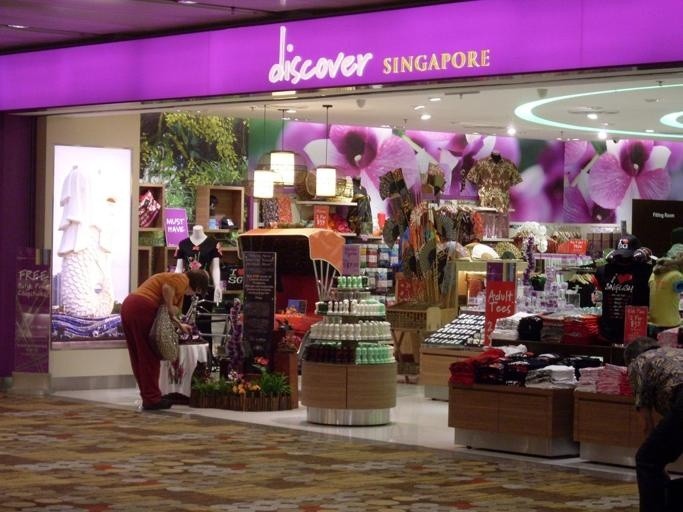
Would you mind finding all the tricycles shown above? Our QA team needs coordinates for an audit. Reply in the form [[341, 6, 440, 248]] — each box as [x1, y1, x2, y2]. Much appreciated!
[[176, 227, 346, 380]]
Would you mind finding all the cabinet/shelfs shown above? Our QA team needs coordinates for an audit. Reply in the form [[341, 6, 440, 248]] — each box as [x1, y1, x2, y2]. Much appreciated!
[[138, 182, 245, 296], [470, 207, 515, 244], [294, 287, 398, 428], [445, 378, 682, 474], [295, 196, 358, 239]]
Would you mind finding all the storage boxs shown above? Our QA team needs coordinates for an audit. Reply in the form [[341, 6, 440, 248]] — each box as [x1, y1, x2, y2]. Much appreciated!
[[357, 242, 399, 289]]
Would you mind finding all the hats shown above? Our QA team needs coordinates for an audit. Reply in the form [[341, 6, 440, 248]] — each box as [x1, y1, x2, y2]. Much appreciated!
[[610, 235, 638, 256], [446, 241, 522, 260]]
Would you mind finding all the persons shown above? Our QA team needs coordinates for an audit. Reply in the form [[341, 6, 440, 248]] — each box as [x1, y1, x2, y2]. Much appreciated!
[[120, 265, 208, 410], [648, 243, 683, 341], [620, 340, 683, 512], [591, 251, 650, 337]]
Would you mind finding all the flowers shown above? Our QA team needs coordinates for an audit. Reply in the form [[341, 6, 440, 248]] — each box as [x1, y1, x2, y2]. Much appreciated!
[[193, 372, 290, 397], [515, 221, 549, 252]]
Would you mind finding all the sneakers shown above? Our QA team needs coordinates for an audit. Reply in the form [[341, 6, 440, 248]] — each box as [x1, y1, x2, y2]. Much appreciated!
[[142, 398, 172, 409]]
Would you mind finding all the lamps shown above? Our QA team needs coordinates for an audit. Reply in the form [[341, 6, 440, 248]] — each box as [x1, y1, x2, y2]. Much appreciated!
[[250, 102, 336, 199]]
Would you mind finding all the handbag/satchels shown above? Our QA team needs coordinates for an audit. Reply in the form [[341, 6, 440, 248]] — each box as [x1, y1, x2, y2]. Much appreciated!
[[147, 304, 179, 363]]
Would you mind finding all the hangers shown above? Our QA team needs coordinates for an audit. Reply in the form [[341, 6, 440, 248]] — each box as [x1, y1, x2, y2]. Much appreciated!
[[544, 221, 581, 245], [569, 264, 598, 286]]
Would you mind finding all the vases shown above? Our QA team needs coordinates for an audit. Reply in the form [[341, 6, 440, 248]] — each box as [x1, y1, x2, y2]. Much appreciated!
[[192, 389, 290, 412]]
